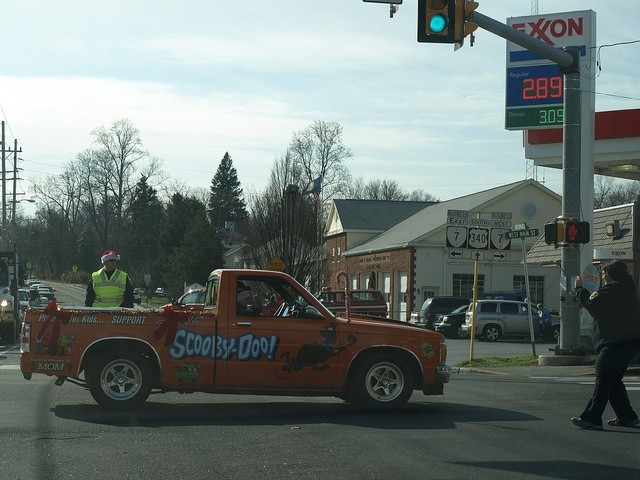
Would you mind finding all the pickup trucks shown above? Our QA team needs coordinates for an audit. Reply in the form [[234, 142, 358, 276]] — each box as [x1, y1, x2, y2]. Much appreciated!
[[20, 269, 451, 410]]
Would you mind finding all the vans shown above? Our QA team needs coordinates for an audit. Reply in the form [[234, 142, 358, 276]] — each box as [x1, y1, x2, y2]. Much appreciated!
[[420, 296, 470, 330], [465, 300, 560, 341], [313, 287, 387, 317], [19, 289, 31, 310]]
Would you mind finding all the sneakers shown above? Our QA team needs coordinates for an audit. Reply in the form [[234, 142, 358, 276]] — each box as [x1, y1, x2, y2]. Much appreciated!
[[571, 417, 603, 431], [607, 418, 640, 428]]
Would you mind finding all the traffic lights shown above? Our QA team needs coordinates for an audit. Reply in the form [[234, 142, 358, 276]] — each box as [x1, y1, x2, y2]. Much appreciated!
[[418, 0, 479, 51], [566, 221, 589, 243], [545, 224, 564, 244]]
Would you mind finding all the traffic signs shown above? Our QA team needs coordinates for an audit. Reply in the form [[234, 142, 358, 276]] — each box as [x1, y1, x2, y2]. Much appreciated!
[[445, 209, 512, 261]]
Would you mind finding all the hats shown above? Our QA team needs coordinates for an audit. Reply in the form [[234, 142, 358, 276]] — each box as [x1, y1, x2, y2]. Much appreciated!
[[101, 249, 120, 263], [236, 281, 250, 294]]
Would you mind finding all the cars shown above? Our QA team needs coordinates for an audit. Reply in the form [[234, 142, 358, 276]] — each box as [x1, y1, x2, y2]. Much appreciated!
[[133, 290, 141, 305], [440, 304, 469, 339], [160, 289, 248, 311]]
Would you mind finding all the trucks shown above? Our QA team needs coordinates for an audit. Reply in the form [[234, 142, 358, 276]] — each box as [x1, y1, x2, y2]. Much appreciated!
[[0, 251, 20, 344]]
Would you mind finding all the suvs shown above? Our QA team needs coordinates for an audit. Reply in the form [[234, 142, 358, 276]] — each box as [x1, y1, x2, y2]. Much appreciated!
[[38, 286, 56, 300]]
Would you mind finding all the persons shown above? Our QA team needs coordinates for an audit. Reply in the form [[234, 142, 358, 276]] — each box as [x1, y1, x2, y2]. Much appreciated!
[[85, 249, 133, 307], [537, 303, 552, 343], [237, 282, 261, 316], [571, 261, 640, 430]]
